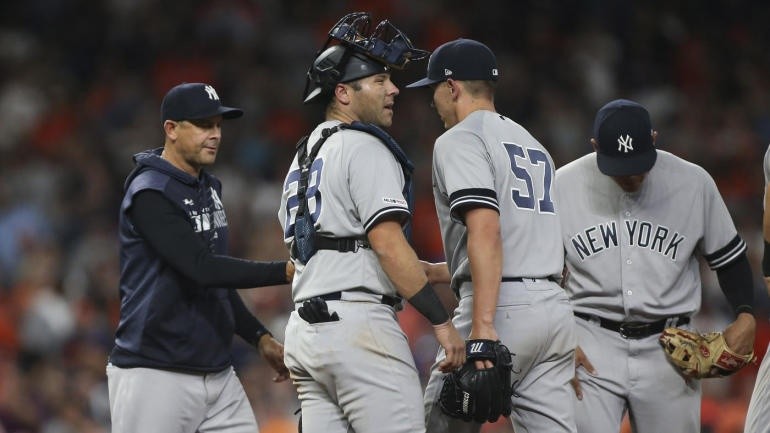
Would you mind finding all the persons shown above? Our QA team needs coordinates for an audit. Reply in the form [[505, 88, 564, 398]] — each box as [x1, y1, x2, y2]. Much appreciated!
[[0, 0, 770, 433], [106, 82, 294, 433]]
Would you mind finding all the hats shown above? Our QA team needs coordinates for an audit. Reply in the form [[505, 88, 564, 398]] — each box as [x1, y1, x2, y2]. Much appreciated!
[[405, 38, 498, 88], [161, 84, 243, 123], [593, 99, 657, 176]]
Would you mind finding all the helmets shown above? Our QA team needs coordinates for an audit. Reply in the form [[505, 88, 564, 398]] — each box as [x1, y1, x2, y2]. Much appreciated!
[[302, 45, 389, 104]]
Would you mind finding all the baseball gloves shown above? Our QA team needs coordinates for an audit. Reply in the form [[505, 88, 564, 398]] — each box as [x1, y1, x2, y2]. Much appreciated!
[[439, 337, 514, 424], [658, 326, 755, 383]]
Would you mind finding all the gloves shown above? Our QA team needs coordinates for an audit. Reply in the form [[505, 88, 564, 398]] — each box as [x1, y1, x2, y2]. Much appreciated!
[[298, 299, 339, 325]]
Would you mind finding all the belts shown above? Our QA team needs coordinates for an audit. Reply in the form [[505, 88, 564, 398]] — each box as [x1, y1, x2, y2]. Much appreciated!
[[321, 292, 401, 306], [455, 276, 556, 300], [574, 311, 690, 340]]
